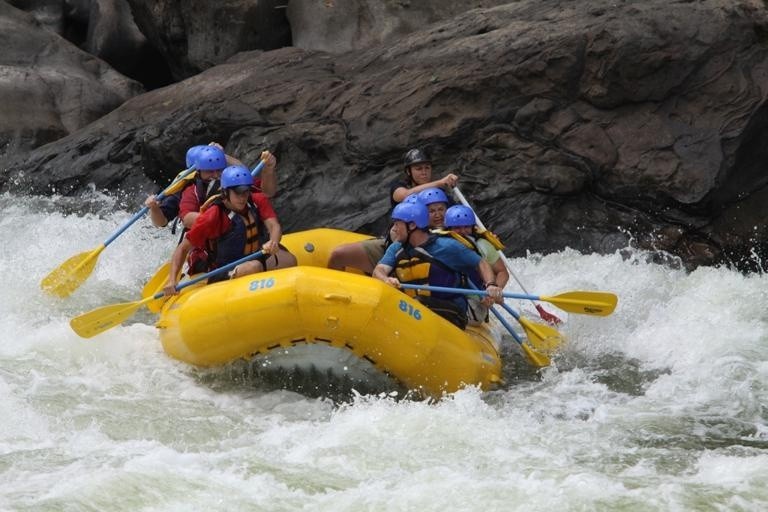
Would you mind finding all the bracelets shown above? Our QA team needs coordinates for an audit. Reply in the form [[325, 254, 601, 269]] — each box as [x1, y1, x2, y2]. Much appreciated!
[[485, 282, 499, 287]]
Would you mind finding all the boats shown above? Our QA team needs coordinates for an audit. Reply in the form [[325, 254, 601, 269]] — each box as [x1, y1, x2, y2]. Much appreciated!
[[146, 216, 512, 401]]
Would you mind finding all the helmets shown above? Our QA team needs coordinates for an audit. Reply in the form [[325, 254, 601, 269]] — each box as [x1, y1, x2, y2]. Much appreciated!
[[444, 205, 476, 228], [221, 165, 254, 189], [404, 148, 431, 167], [392, 203, 429, 228], [186, 144, 227, 171], [401, 188, 448, 205]]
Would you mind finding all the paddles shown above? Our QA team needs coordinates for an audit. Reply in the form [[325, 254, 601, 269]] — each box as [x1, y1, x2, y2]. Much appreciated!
[[399, 283, 616, 316], [40, 165, 197, 299], [452, 186, 566, 368], [70, 251, 268, 338], [142, 160, 266, 313]]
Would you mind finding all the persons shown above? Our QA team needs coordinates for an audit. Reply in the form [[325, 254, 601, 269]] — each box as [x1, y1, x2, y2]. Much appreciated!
[[441, 205, 509, 307], [146, 141, 246, 228], [384, 149, 458, 251], [164, 166, 301, 296], [373, 202, 503, 330], [179, 147, 278, 277], [420, 188, 451, 236]]
